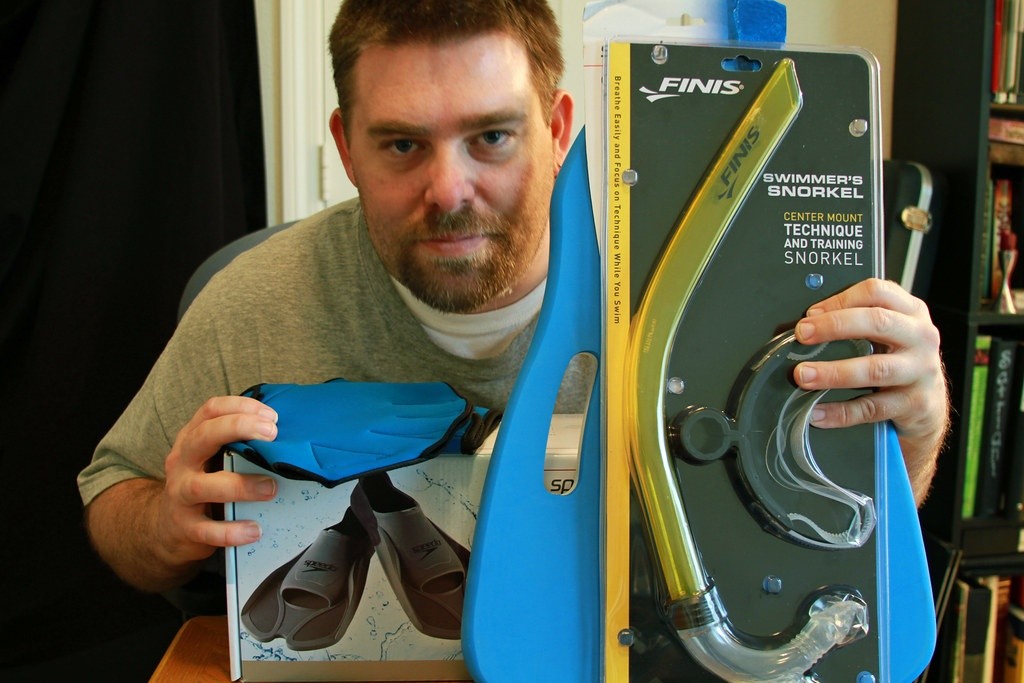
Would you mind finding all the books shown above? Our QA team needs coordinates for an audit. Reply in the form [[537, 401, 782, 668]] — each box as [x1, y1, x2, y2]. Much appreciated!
[[956, 2, 1024, 683]]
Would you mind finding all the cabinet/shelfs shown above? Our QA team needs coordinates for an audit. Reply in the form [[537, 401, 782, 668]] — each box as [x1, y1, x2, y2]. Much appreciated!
[[894, 0, 1024, 575]]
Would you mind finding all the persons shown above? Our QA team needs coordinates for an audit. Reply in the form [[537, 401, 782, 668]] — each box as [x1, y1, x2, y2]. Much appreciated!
[[78, 1, 952, 683]]
